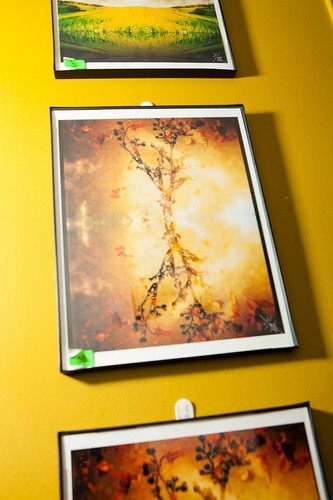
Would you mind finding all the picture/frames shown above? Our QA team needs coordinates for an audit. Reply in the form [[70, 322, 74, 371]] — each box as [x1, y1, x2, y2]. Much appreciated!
[[58, 400, 328, 500], [50, 103, 300, 373], [49, 0, 237, 79]]
